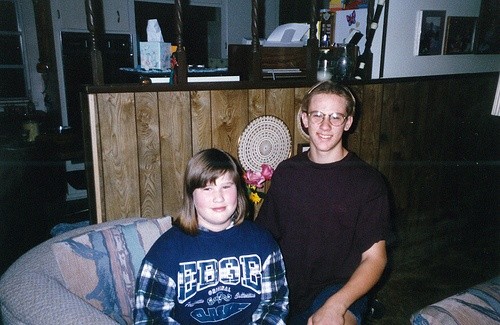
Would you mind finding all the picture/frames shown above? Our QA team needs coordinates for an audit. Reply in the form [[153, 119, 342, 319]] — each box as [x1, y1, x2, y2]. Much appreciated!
[[413, 9, 445, 56], [442, 14, 477, 55], [474, 0, 500, 55]]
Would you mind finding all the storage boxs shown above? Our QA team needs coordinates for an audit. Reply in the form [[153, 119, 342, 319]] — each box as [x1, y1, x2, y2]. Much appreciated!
[[227, 43, 304, 72]]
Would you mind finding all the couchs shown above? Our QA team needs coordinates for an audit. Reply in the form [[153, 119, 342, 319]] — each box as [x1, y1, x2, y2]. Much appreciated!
[[0, 217, 175, 325]]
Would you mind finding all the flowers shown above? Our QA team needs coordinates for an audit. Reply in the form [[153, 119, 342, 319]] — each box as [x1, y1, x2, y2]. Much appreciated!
[[242, 163, 272, 203]]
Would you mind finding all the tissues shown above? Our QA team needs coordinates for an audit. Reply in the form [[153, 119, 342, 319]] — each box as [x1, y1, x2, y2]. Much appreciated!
[[138, 18, 172, 70]]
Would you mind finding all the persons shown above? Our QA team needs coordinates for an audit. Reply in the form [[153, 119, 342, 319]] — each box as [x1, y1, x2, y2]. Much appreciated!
[[255, 83, 391, 325], [135, 149, 289, 325]]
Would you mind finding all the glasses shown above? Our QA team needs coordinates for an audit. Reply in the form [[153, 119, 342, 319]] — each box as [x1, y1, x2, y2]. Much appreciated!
[[308, 110, 348, 126]]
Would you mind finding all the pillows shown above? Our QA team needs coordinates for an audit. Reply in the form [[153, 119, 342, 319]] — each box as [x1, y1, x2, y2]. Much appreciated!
[[54, 214, 174, 324]]
